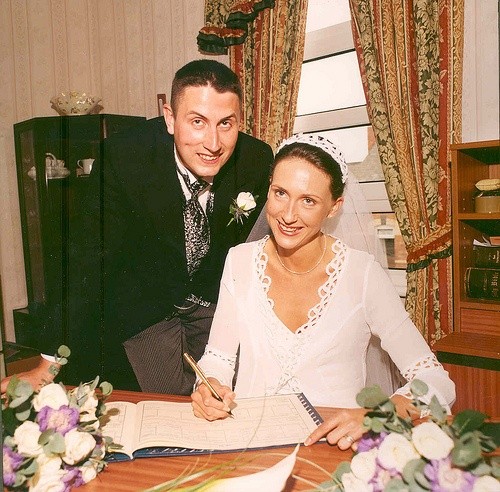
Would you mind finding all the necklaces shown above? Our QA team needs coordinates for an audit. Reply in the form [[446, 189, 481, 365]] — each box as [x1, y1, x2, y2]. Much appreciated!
[[275, 235, 327, 275]]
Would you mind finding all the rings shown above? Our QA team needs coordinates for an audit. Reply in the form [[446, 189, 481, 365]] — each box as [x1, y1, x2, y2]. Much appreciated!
[[344, 433, 355, 444]]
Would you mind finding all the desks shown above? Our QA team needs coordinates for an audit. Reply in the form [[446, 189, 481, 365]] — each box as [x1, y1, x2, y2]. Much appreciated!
[[65, 385, 500, 492]]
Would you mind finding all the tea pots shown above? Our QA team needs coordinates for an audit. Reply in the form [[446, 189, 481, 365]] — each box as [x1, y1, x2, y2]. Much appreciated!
[[44, 153, 57, 168]]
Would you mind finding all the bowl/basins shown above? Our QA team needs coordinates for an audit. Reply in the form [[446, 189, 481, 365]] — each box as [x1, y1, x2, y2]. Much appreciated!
[[50, 91, 102, 116]]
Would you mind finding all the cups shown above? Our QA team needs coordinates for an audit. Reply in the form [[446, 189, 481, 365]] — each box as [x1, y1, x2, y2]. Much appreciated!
[[52, 160, 65, 167], [27, 167, 70, 179], [77, 159, 95, 175]]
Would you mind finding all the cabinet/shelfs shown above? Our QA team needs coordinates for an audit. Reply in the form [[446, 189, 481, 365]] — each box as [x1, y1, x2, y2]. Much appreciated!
[[14, 114, 165, 385], [431, 141, 500, 423]]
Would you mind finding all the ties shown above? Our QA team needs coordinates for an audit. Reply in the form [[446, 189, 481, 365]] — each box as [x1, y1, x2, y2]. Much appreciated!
[[175, 163, 211, 282]]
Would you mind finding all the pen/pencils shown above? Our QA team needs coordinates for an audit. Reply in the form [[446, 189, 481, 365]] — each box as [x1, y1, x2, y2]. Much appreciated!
[[182, 351, 235, 417]]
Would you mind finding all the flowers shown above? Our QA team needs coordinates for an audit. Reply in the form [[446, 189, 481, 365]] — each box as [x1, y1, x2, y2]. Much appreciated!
[[0, 345, 123, 492], [315, 379, 500, 492], [225, 188, 259, 227]]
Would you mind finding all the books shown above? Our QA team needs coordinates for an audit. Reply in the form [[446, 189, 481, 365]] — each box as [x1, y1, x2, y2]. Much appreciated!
[[98, 392, 329, 461], [465, 237, 500, 301]]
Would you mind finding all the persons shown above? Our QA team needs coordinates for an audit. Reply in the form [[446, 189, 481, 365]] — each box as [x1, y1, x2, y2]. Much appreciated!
[[0, 58, 274, 407], [192, 134, 455, 451]]
